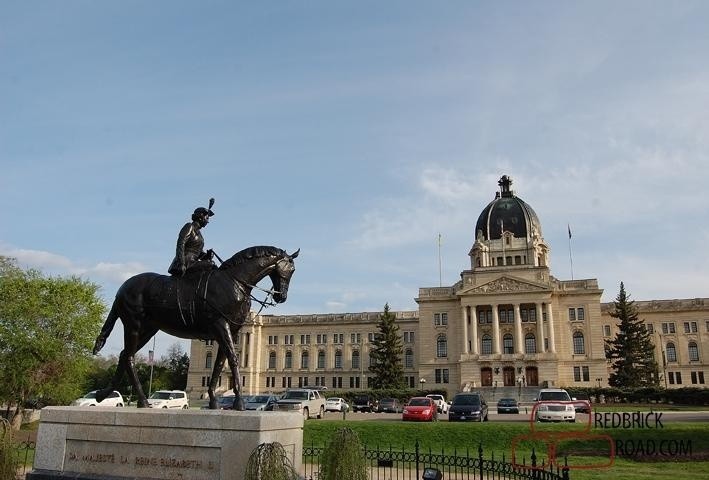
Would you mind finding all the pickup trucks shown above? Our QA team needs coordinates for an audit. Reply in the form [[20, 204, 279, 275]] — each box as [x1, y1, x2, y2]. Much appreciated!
[[571, 394, 591, 415]]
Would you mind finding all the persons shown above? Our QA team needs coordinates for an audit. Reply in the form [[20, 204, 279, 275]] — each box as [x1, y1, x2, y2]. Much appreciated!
[[167, 196, 218, 275]]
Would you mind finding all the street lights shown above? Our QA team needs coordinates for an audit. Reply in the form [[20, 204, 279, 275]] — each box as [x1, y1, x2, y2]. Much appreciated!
[[596, 377, 602, 388], [419, 377, 426, 389]]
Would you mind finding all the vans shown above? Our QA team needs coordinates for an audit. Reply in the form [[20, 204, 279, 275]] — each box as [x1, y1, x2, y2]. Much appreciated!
[[533, 387, 575, 423]]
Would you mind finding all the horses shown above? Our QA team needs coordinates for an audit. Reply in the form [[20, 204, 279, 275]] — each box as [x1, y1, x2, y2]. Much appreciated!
[[92, 246, 300, 411]]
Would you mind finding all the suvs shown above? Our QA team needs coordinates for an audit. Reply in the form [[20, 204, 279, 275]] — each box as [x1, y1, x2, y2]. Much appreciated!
[[273, 387, 327, 419], [74, 389, 125, 407]]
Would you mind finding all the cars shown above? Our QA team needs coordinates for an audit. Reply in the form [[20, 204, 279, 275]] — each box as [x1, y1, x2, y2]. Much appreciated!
[[143, 389, 189, 411], [203, 387, 520, 423]]
